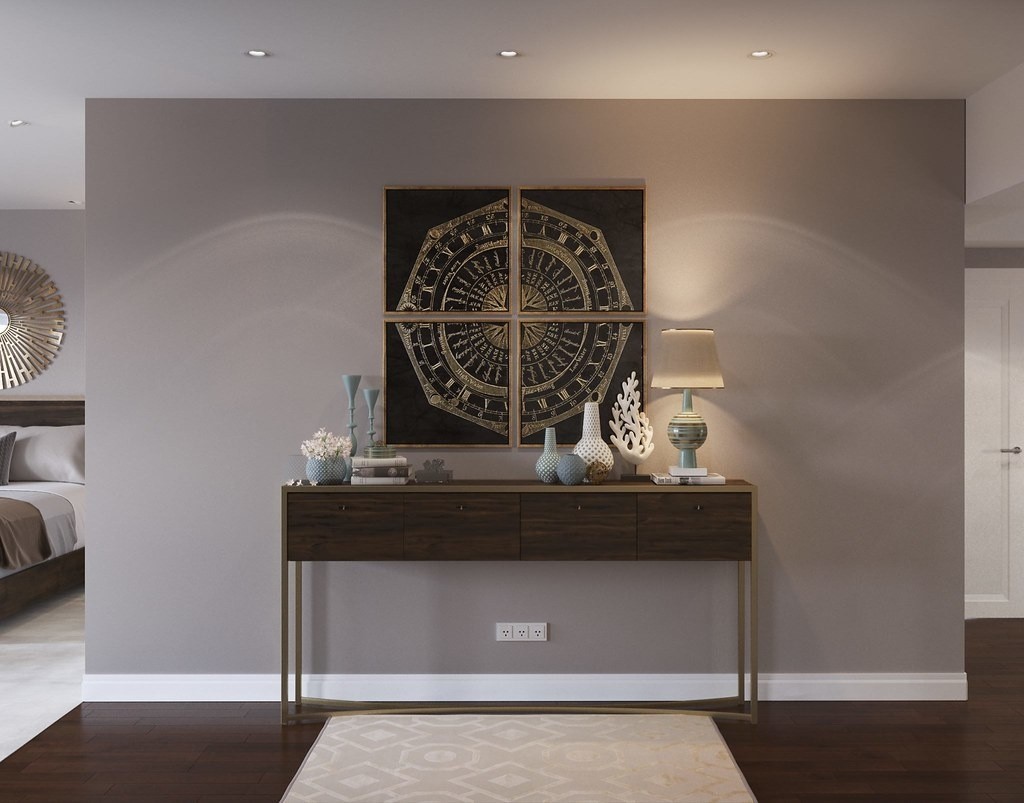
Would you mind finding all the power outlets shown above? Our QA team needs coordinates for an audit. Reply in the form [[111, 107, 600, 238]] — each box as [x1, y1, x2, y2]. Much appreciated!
[[495, 622, 548, 641]]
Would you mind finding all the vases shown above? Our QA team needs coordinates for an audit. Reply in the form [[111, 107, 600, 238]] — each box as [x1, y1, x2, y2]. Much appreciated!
[[363, 388, 380, 448], [341, 374, 362, 481], [557, 454, 587, 484], [572, 401, 615, 483], [535, 428, 562, 483], [306, 455, 347, 485]]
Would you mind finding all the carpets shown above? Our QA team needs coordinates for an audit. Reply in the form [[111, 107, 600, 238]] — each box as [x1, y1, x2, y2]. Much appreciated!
[[280, 714, 761, 803], [0, 581, 86, 763]]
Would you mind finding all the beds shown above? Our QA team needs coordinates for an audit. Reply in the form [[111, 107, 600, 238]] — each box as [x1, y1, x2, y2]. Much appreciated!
[[0, 394, 86, 620]]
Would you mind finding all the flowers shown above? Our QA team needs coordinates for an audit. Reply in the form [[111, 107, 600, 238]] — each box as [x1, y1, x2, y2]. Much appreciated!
[[309, 480, 318, 486], [286, 478, 296, 486], [301, 427, 353, 460], [296, 480, 303, 486]]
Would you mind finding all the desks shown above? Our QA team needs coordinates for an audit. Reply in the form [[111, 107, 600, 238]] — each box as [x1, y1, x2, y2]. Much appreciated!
[[280, 479, 760, 725]]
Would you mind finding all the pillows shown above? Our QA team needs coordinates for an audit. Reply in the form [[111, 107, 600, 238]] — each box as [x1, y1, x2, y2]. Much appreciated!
[[0, 430, 17, 486], [0, 424, 85, 485]]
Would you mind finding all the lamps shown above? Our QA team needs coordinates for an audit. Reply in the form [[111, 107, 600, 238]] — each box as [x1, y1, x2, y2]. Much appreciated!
[[650, 329, 726, 486]]
